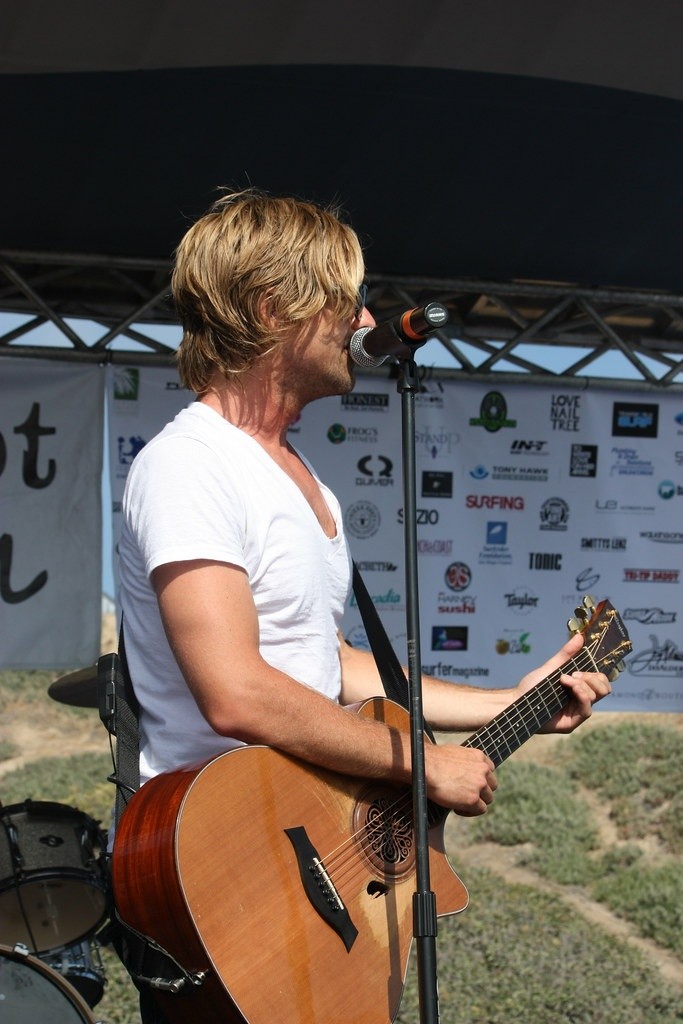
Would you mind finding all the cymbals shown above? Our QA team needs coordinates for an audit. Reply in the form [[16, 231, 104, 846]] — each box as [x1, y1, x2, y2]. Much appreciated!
[[49, 663, 100, 709]]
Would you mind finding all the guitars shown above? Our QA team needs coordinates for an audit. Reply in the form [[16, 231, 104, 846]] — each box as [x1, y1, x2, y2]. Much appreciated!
[[111, 594, 633, 1024]]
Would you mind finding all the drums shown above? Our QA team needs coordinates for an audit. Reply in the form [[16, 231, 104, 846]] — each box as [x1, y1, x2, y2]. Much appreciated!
[[37, 938, 108, 1011], [0, 941, 99, 1024], [0, 795, 113, 957]]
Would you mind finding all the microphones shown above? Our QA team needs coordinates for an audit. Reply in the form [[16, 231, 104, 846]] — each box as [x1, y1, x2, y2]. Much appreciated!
[[349, 302, 450, 368]]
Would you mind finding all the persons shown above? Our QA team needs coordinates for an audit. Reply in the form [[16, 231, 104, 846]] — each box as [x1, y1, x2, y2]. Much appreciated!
[[113, 189, 612, 1024]]
